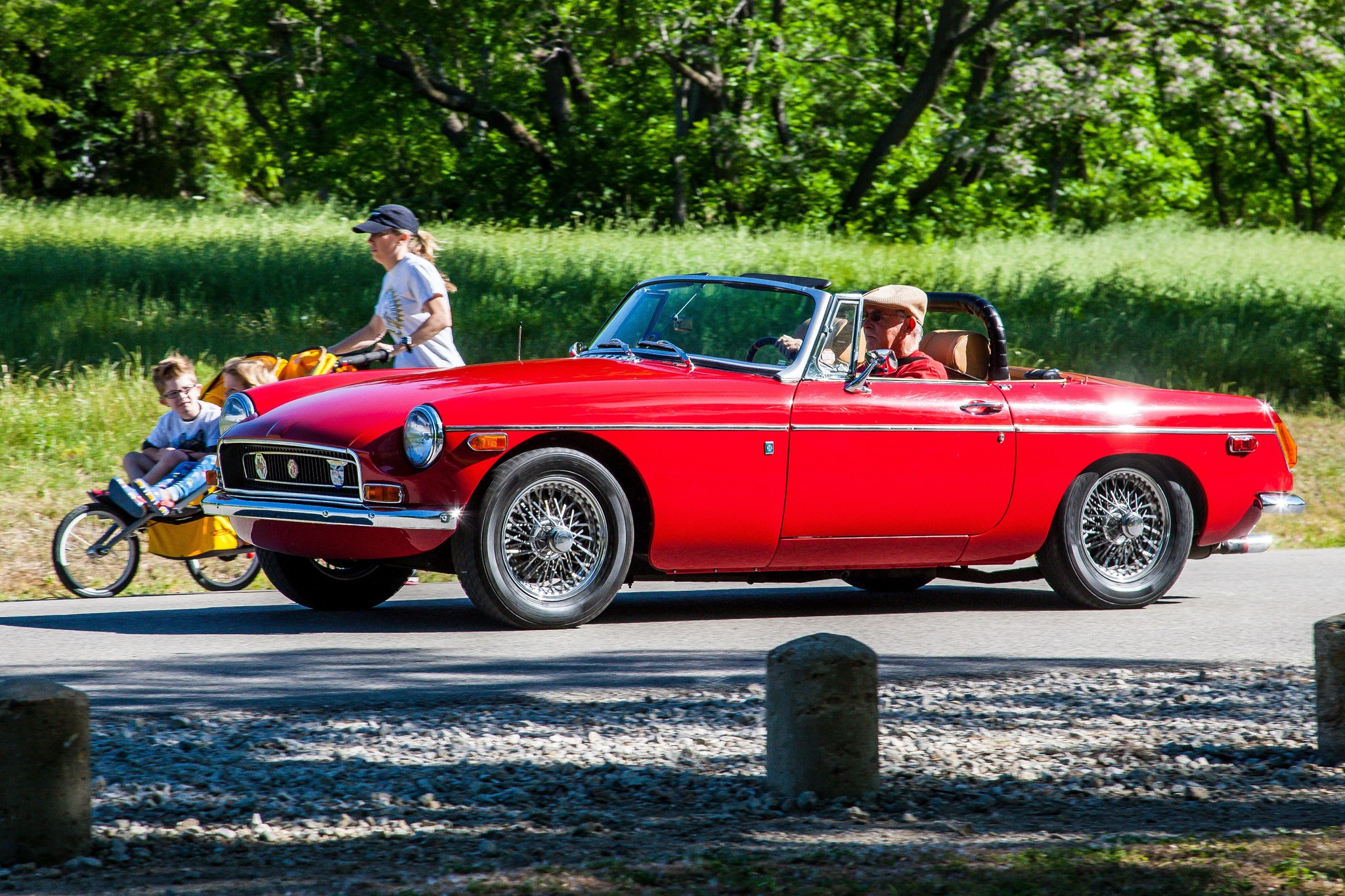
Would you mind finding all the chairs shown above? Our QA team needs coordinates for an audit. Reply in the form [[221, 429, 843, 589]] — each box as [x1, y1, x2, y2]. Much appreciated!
[[790, 317, 867, 370], [918, 327, 990, 381]]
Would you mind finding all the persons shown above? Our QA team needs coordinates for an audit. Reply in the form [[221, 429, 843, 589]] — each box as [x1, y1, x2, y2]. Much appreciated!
[[327, 204, 468, 583], [85, 355, 276, 523], [775, 284, 947, 380]]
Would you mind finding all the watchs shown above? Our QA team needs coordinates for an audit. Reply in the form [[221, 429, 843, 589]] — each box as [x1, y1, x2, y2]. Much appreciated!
[[399, 336, 413, 355]]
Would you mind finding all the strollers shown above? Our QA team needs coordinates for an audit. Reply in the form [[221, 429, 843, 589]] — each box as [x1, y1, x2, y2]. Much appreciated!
[[52, 347, 390, 598]]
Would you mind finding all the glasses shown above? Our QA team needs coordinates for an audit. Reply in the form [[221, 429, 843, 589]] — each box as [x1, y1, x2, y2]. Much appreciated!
[[865, 310, 908, 323], [161, 384, 195, 400]]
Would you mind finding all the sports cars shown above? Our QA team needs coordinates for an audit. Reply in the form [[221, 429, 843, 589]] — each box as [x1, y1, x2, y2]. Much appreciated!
[[200, 271, 1308, 632]]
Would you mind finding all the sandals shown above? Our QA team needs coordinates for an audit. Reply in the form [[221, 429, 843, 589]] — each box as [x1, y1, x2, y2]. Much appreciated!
[[109, 477, 149, 518], [134, 478, 175, 517]]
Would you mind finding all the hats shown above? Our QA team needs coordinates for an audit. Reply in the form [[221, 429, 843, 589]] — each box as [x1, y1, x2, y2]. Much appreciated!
[[352, 204, 418, 236], [861, 284, 928, 325]]
